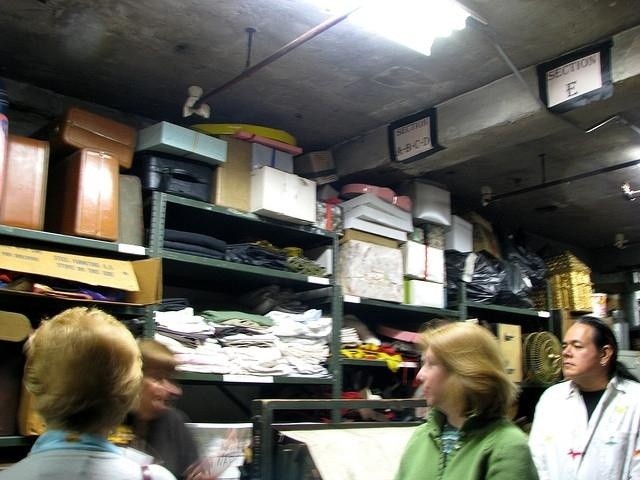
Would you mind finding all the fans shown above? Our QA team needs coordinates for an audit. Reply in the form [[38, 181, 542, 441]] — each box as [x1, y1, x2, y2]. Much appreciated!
[[522, 332, 562, 386]]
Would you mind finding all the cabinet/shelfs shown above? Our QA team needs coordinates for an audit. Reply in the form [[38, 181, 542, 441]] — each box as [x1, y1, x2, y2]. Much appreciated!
[[2, 224, 151, 450], [339, 238, 461, 424], [152, 192, 338, 426], [463, 279, 554, 419]]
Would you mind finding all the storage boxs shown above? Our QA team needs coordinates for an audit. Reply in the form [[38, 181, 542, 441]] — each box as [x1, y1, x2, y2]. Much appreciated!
[[340, 193, 412, 223], [344, 218, 407, 241], [339, 239, 405, 302], [413, 179, 452, 228], [445, 215, 474, 254], [2, 135, 52, 230], [58, 149, 119, 241], [140, 121, 228, 163], [425, 222, 444, 247], [2, 114, 8, 187], [213, 135, 295, 209], [404, 278, 446, 309], [50, 110, 138, 169], [142, 151, 208, 200], [466, 211, 500, 257], [344, 206, 413, 232], [251, 167, 315, 220], [410, 226, 425, 241], [398, 240, 446, 282], [341, 228, 398, 249]]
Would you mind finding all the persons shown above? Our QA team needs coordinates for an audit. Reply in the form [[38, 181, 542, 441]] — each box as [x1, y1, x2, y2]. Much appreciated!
[[0, 306, 206, 480], [395, 317, 640, 479]]
